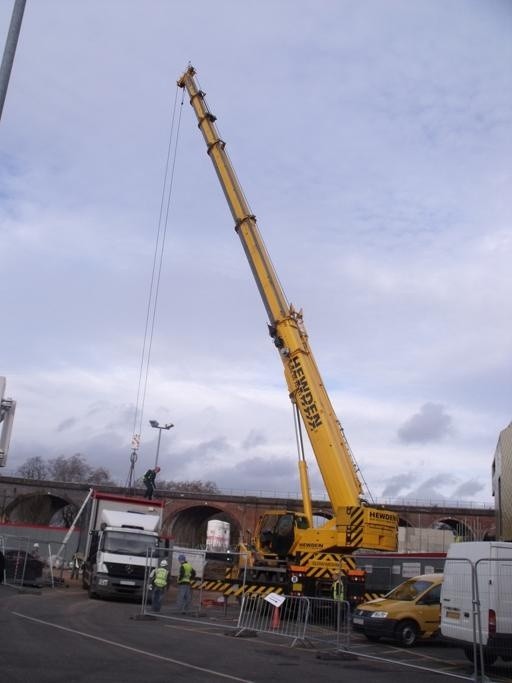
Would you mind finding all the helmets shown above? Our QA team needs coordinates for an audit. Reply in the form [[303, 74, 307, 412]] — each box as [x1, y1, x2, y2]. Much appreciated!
[[159, 558, 168, 567]]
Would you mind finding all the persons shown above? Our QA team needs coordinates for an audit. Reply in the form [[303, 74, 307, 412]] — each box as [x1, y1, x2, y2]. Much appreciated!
[[149, 559, 170, 612], [70, 559, 82, 579], [330, 572, 345, 631], [144, 467, 161, 501], [175, 555, 196, 609]]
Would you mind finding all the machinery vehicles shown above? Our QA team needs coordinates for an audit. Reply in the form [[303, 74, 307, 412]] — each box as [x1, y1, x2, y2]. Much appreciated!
[[176, 63, 398, 627]]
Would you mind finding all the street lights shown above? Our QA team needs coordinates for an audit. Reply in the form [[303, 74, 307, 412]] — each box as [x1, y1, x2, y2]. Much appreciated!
[[149, 418, 175, 489]]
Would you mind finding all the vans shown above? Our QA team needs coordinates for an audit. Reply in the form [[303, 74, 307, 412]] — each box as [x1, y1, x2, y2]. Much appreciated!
[[352, 572, 444, 645]]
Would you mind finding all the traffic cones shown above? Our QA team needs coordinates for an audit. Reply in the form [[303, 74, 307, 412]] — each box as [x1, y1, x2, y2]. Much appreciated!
[[266, 603, 282, 630]]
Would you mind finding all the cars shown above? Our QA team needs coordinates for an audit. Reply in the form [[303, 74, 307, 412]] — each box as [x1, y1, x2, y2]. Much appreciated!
[[0, 545, 44, 582]]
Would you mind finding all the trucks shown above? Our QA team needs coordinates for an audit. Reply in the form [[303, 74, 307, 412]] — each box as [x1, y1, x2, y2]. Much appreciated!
[[77, 490, 166, 602], [439, 540, 512, 666]]
